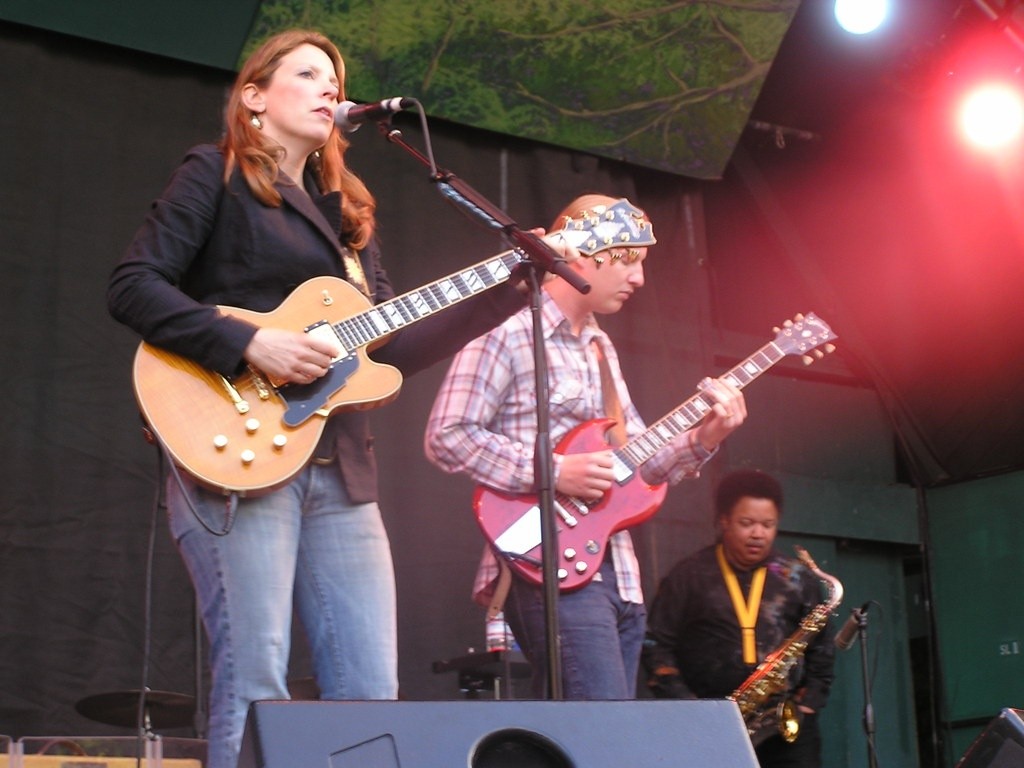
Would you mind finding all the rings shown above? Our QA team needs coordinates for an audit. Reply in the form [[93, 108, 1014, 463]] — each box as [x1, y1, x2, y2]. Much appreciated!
[[728, 411, 734, 419]]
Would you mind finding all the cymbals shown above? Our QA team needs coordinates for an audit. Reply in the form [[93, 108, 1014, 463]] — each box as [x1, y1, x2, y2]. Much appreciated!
[[73, 688, 199, 729]]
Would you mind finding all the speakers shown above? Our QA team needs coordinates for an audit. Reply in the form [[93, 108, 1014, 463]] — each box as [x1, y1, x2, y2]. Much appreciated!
[[957, 707, 1024, 768], [237, 701, 762, 768]]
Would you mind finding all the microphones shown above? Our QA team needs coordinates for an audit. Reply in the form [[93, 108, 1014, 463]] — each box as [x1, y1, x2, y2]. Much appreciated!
[[333, 98, 418, 133], [835, 603, 870, 651]]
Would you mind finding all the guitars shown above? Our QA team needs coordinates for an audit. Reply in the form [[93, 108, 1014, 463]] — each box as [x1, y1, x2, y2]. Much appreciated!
[[131, 193, 661, 501], [470, 309, 841, 594]]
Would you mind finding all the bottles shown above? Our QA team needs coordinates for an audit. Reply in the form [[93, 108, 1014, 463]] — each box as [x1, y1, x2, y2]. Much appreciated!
[[486, 611, 513, 653]]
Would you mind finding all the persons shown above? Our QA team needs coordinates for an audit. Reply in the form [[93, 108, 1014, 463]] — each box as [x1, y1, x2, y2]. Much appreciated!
[[640, 468, 837, 768], [420, 195, 746, 701], [106, 27, 579, 768]]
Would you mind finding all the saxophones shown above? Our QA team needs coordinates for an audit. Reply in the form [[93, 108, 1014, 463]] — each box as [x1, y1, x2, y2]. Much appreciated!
[[724, 542, 846, 748]]
